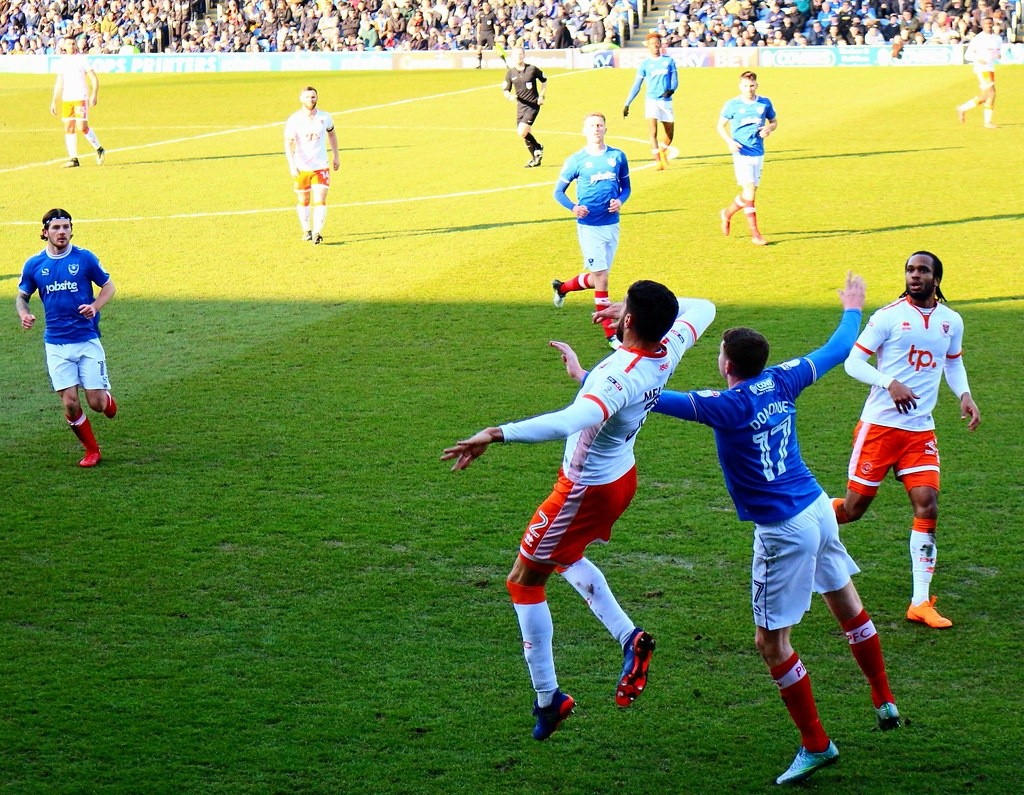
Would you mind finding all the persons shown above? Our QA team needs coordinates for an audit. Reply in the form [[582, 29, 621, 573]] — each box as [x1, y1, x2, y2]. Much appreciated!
[[551, 113, 631, 350], [622, 32, 678, 171], [15, 209, 116, 466], [502, 46, 547, 167], [831, 250, 982, 627], [50, 37, 105, 167], [955, 17, 1003, 128], [716, 71, 778, 246], [441, 280, 717, 740], [284, 87, 339, 245], [549, 271, 902, 786], [0, 0, 1023, 56]]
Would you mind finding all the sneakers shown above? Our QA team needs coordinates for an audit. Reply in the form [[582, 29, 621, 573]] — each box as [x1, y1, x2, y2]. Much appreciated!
[[721, 210, 730, 235], [653, 150, 668, 171], [776, 739, 840, 785], [64, 158, 79, 167], [873, 702, 900, 727], [906, 596, 953, 628], [104, 391, 117, 418], [615, 629, 656, 706], [525, 144, 543, 167], [303, 231, 323, 244], [608, 333, 623, 350], [533, 690, 574, 741], [553, 280, 566, 308], [80, 448, 101, 467], [752, 236, 768, 245], [97, 147, 105, 165]]
